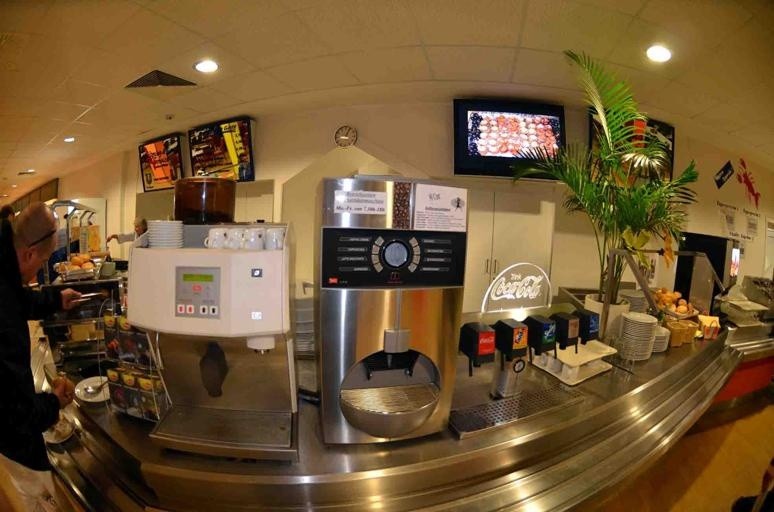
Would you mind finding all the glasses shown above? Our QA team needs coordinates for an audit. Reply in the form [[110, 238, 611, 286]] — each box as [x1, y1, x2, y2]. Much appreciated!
[[22, 206, 62, 248]]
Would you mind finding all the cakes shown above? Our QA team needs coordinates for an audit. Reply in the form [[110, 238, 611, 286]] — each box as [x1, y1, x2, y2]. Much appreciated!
[[477, 115, 560, 159]]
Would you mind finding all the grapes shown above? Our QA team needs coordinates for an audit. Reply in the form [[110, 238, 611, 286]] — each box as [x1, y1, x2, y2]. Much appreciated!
[[467, 112, 483, 157]]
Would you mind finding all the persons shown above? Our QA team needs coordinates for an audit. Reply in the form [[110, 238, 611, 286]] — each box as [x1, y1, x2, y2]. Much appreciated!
[[107, 217, 149, 248], [0, 202, 83, 471]]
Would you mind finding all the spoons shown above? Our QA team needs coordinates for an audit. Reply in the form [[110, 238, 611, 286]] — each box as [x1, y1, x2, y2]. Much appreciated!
[[86, 378, 111, 395]]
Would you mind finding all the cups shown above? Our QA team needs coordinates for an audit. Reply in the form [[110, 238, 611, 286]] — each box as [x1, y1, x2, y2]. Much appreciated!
[[203, 227, 284, 249]]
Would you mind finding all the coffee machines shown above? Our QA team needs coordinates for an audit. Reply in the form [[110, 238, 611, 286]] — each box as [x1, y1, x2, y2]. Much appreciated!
[[125, 178, 297, 465], [317, 175, 464, 446]]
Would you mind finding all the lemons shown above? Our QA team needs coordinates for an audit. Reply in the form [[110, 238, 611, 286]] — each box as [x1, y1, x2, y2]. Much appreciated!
[[655, 287, 694, 313], [59, 253, 93, 271]]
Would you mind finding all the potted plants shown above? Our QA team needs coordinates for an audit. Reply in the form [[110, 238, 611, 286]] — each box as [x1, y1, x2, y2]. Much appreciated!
[[510, 49, 701, 340]]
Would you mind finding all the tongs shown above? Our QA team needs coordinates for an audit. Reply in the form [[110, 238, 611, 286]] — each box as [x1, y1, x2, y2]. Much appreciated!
[[68, 292, 105, 303]]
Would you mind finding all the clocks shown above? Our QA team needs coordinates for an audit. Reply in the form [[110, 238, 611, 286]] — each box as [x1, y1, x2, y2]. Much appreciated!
[[334, 125, 357, 148]]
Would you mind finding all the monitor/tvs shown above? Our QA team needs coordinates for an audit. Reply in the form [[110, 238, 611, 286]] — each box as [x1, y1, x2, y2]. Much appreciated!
[[453, 98, 566, 180]]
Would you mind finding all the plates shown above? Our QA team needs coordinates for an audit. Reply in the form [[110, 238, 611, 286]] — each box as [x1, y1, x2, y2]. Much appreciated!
[[42, 413, 76, 445], [652, 326, 671, 353], [618, 311, 658, 361], [147, 220, 184, 248], [74, 376, 111, 404], [619, 288, 648, 314]]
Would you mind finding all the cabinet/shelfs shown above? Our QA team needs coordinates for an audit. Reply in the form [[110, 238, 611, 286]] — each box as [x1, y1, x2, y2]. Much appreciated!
[[428, 178, 555, 313]]
